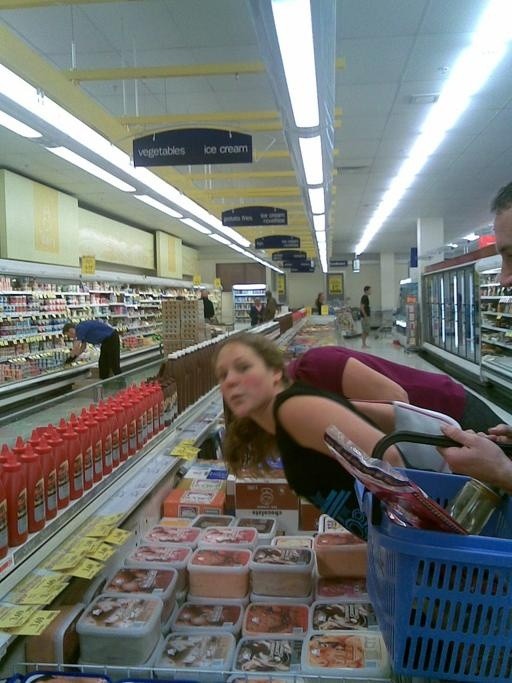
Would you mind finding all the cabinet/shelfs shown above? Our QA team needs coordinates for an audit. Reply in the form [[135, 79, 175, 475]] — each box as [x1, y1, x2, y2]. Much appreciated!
[[474, 243, 512, 395], [0, 257, 224, 429]]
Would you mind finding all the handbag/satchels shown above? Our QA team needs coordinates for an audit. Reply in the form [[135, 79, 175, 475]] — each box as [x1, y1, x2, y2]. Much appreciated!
[[388, 400, 463, 474]]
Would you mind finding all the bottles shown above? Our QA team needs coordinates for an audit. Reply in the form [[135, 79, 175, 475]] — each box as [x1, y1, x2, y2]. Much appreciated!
[[449, 479, 502, 539], [0, 374, 178, 558]]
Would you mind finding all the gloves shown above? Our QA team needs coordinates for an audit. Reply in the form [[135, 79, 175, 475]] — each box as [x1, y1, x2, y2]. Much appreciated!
[[65, 355, 78, 364]]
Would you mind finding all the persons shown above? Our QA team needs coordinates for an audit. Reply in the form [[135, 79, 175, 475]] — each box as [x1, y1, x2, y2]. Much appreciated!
[[212, 335, 511, 540], [197, 289, 215, 322], [437, 182, 511, 490], [249, 291, 278, 326], [360, 286, 372, 349], [63, 320, 127, 391], [315, 292, 326, 315]]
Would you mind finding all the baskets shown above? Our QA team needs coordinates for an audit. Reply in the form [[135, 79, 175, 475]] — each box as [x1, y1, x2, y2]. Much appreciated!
[[353, 432, 510, 681]]
